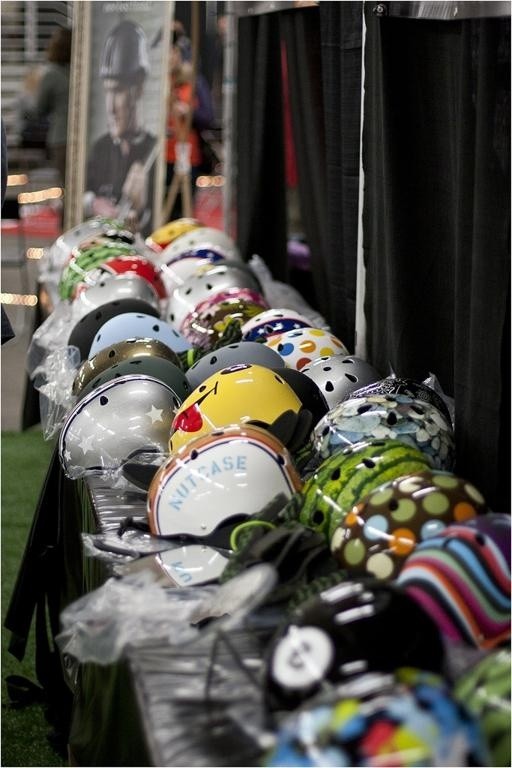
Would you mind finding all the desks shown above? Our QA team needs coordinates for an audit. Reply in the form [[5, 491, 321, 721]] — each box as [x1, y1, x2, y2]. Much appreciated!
[[33, 250, 512, 764]]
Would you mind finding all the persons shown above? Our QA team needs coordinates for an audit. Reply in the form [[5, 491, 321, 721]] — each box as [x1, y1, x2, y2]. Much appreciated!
[[82, 18, 157, 230], [14, 22, 72, 175], [167, 36, 214, 219]]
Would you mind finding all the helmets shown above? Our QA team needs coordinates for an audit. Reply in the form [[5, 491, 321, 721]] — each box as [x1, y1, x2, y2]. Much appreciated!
[[45, 218, 511, 767], [99, 20, 150, 86]]
